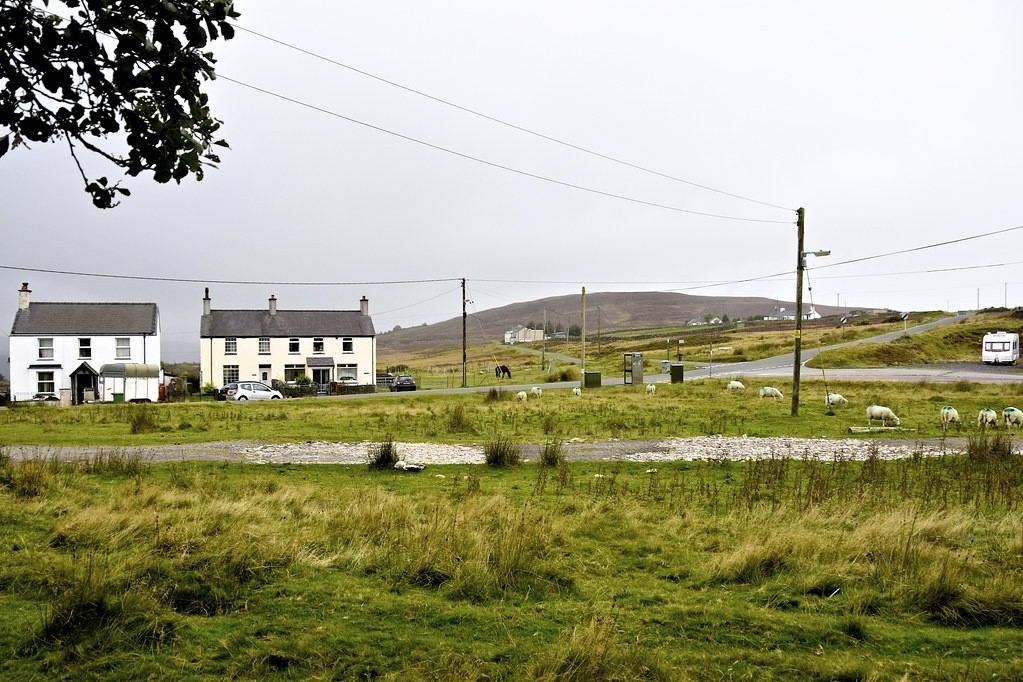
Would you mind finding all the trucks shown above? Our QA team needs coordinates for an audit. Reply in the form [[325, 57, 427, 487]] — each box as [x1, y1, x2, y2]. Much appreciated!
[[982, 332, 1019, 364]]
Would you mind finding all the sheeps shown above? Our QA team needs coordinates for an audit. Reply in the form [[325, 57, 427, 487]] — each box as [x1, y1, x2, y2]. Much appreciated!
[[516, 390, 528, 403], [824, 392, 849, 409], [976, 407, 1000, 431], [758, 385, 783, 402], [1002, 406, 1023, 431], [645, 383, 656, 397], [940, 406, 963, 432], [572, 385, 582, 396], [727, 380, 746, 391], [865, 404, 900, 427], [531, 386, 543, 399]]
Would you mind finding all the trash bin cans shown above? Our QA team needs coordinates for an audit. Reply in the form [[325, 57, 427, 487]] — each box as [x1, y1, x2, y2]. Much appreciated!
[[330, 382, 338, 396], [111, 392, 125, 405], [669, 364, 684, 384], [584, 371, 602, 388]]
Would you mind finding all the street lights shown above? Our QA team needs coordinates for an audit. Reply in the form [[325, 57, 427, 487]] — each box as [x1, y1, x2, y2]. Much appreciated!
[[790, 250, 831, 417]]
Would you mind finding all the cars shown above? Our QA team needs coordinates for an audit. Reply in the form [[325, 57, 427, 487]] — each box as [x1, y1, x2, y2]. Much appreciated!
[[219, 383, 238, 400], [226, 381, 283, 401], [32, 392, 59, 401]]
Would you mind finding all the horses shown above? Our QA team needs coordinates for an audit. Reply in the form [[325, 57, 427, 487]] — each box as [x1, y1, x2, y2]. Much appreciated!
[[495, 365, 512, 379]]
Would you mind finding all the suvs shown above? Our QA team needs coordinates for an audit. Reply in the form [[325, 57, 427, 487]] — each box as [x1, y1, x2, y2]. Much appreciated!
[[389, 375, 417, 392]]
